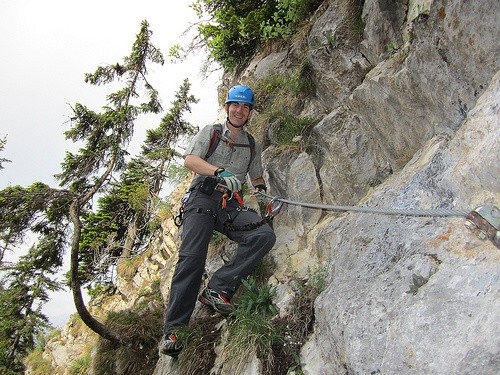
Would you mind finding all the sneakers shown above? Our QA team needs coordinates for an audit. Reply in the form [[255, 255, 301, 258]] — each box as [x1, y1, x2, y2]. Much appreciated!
[[160, 333, 184, 357], [197, 283, 237, 318]]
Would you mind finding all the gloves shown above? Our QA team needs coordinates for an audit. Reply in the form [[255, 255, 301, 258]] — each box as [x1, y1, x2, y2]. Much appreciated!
[[219, 169, 243, 193], [254, 185, 267, 199]]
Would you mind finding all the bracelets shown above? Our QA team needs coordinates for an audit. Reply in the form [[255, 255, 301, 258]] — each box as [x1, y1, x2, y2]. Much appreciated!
[[255, 184, 267, 193]]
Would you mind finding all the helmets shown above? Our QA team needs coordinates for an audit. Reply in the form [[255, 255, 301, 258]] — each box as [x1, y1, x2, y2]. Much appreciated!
[[224, 85, 256, 105]]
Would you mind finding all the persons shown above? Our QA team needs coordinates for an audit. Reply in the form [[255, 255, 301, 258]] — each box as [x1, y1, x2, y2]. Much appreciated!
[[161, 85, 276, 356]]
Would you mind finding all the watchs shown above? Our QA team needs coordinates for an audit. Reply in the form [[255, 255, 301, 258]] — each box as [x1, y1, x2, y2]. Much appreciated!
[[214, 168, 225, 177]]
